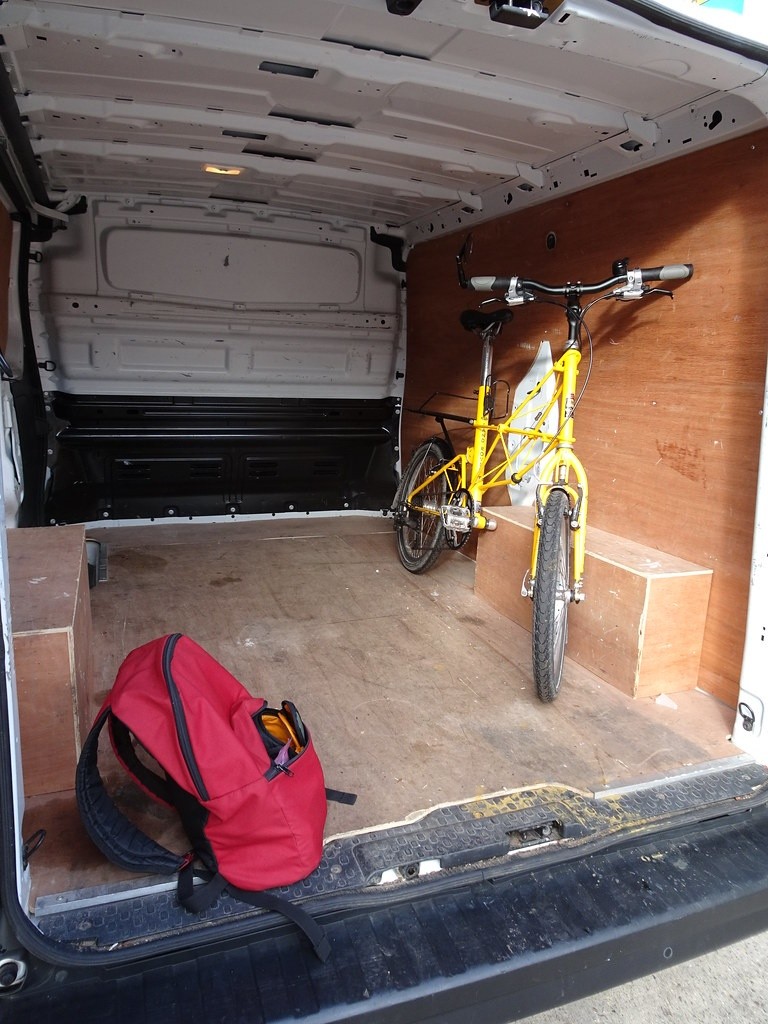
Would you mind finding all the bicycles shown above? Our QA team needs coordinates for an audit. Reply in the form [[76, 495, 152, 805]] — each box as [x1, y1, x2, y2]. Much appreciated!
[[381, 254, 695, 709]]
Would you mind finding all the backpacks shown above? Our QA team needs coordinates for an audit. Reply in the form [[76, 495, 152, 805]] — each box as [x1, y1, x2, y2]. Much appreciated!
[[75, 631, 326, 912]]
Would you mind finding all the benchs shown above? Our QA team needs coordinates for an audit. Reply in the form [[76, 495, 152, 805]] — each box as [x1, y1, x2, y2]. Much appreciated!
[[474, 506, 714, 700], [5, 525, 95, 797]]
